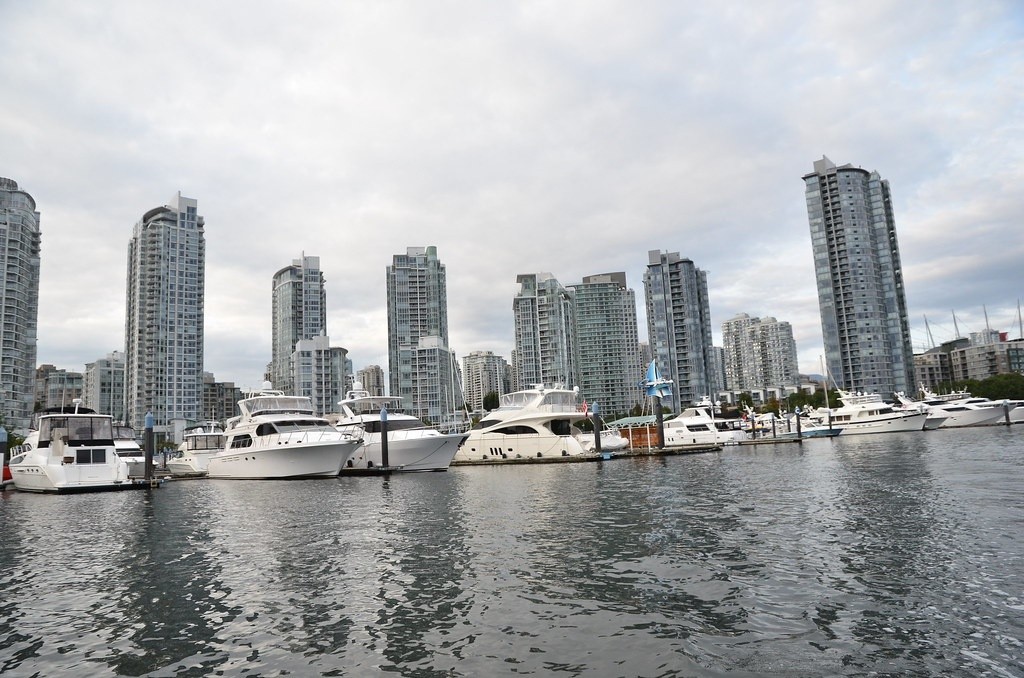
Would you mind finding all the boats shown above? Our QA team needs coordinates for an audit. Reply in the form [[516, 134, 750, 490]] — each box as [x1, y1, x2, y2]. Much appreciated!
[[752, 381, 1024, 435], [7, 410, 160, 495], [328, 373, 470, 472], [451, 383, 632, 463], [166, 395, 362, 479], [663, 407, 749, 447]]
